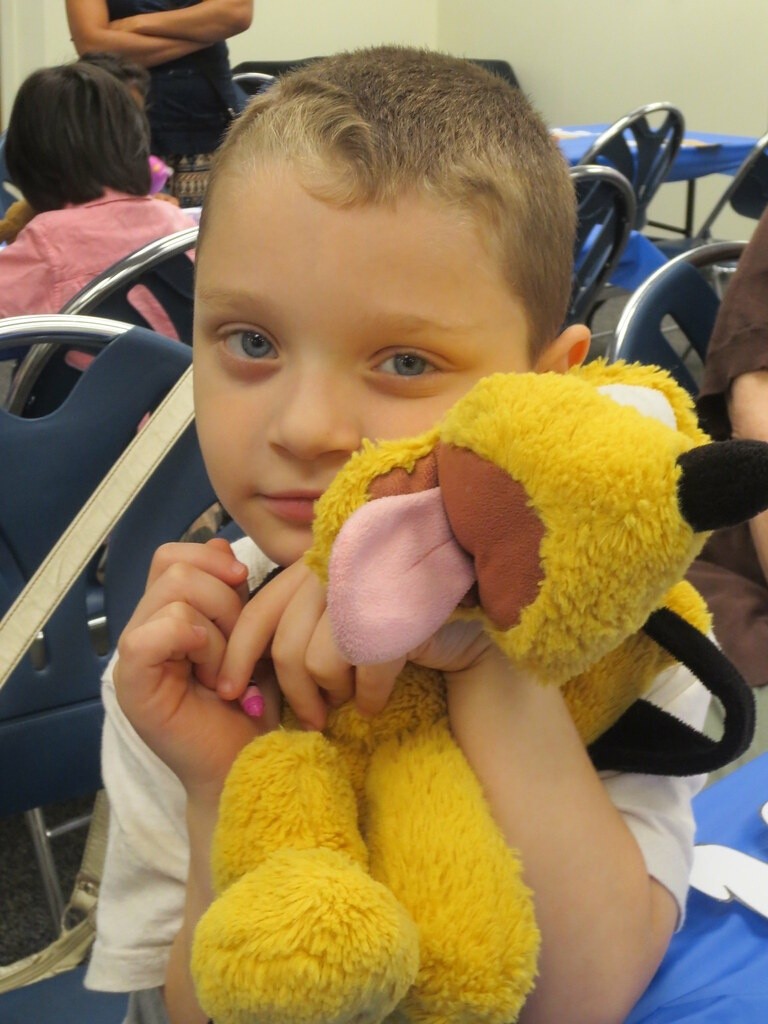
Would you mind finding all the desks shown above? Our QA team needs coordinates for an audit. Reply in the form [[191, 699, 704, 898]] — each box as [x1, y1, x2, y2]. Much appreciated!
[[548, 123, 767, 239]]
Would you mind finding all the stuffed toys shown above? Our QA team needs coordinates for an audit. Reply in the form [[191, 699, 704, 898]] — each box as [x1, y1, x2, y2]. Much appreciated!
[[190, 351, 768, 1024]]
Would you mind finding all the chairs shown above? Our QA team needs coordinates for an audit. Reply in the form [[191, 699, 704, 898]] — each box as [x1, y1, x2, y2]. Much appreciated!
[[0, 58, 768, 1024]]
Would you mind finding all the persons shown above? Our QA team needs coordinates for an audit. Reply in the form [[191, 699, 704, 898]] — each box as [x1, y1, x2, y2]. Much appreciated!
[[688, 205, 768, 688], [0, 0, 256, 572], [87, 42, 712, 1024]]
